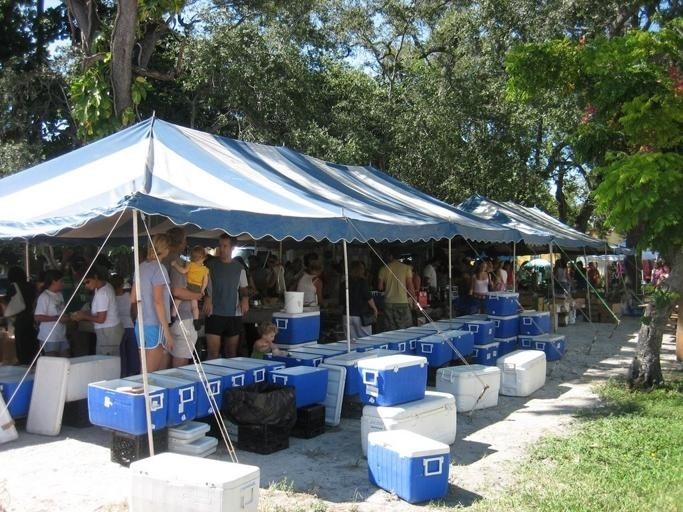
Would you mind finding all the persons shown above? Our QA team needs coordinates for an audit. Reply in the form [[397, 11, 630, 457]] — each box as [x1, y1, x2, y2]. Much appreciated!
[[374, 246, 414, 330], [70, 272, 122, 356], [33, 270, 70, 362], [0, 228, 671, 373], [133, 232, 170, 375], [249, 320, 276, 360], [162, 228, 199, 369], [172, 247, 209, 331], [203, 234, 251, 359]]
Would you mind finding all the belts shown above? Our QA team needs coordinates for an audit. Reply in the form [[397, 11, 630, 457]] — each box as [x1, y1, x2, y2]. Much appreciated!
[[473, 293, 485, 300]]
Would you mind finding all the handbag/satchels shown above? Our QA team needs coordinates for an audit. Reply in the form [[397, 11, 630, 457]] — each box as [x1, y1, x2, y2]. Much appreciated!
[[359, 310, 378, 326], [5, 282, 27, 318]]
